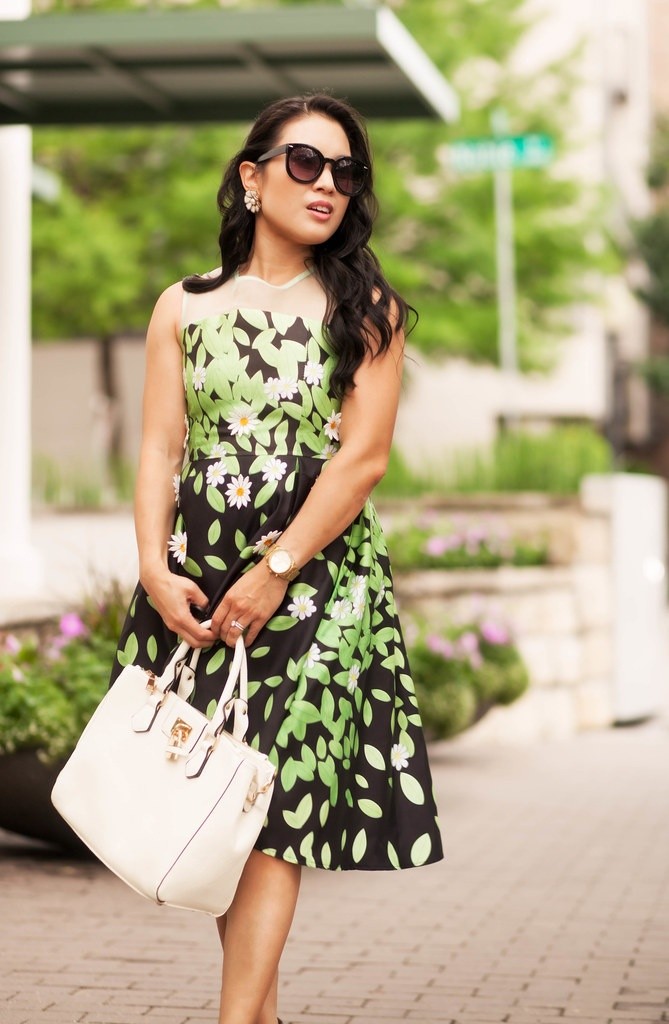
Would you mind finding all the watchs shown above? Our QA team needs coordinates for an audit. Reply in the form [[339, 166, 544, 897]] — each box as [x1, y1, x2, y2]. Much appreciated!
[[264, 541, 300, 583]]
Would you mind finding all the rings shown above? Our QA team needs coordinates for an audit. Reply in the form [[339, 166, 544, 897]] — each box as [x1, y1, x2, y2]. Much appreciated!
[[231, 621, 245, 631]]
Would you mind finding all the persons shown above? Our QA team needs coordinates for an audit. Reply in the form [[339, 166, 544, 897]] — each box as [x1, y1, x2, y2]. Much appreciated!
[[109, 91, 446, 1024]]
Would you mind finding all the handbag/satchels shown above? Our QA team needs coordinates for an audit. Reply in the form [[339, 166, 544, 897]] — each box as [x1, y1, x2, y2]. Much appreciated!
[[52, 615, 278, 920]]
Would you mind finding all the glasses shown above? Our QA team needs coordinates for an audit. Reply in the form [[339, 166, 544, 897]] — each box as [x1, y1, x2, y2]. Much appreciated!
[[256, 143, 372, 197]]
